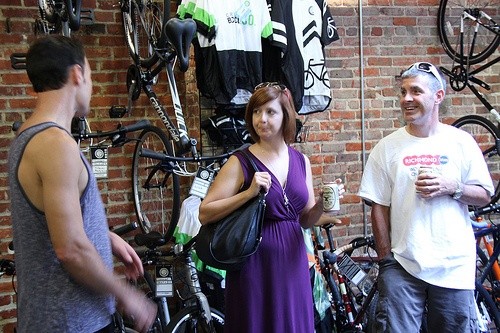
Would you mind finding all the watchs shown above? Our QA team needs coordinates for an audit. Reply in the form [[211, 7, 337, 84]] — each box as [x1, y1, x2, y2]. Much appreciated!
[[452, 182, 464, 200]]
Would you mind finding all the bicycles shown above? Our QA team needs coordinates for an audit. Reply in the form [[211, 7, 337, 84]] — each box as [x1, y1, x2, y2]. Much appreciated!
[[108, 0, 253, 247], [10, 0, 151, 156], [310, 213, 382, 333], [436, 0, 500, 333], [112, 221, 227, 333]]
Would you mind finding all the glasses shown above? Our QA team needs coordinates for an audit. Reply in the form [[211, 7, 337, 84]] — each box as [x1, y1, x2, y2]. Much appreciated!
[[406, 62, 445, 90], [254, 82, 294, 109]]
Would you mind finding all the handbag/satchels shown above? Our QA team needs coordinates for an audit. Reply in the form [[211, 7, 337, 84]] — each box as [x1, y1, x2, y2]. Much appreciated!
[[196, 149, 265, 267]]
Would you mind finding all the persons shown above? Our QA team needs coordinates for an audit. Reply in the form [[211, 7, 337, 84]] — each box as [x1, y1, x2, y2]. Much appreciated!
[[357, 62, 495, 333], [6, 35, 158, 333], [198, 81, 345, 333]]
